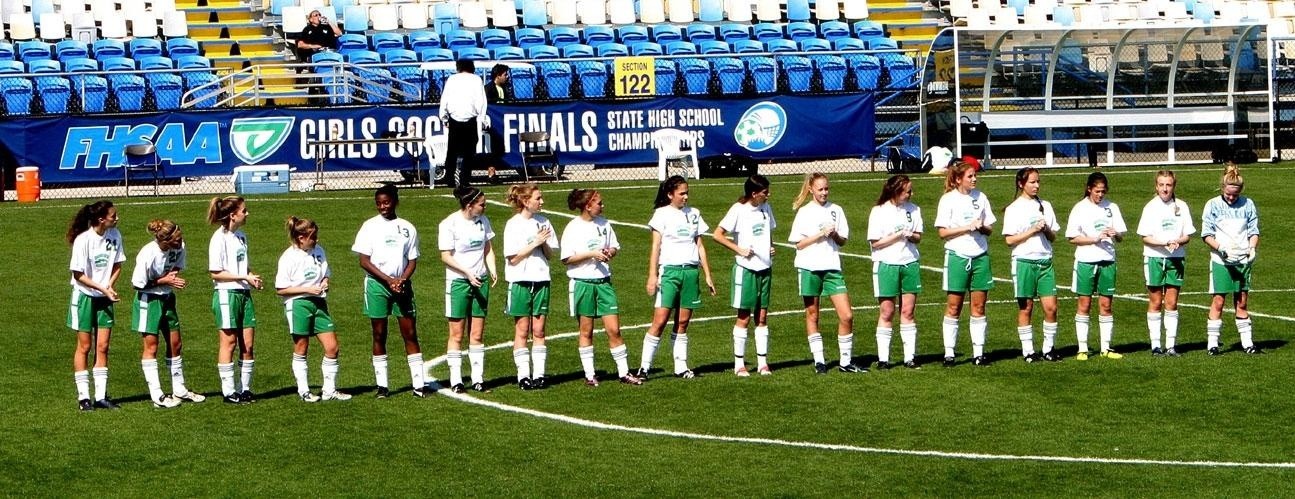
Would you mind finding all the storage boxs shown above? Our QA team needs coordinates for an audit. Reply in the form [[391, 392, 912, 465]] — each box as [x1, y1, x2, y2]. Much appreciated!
[[229, 163, 298, 195]]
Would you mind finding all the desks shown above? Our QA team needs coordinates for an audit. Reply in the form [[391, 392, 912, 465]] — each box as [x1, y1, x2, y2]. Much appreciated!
[[305, 134, 426, 192]]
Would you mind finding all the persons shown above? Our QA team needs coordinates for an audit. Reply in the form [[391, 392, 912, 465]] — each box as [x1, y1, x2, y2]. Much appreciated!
[[1135, 169, 1197, 358], [999, 166, 1066, 365], [710, 173, 779, 379], [64, 199, 127, 412], [436, 183, 500, 396], [272, 216, 355, 405], [438, 57, 489, 191], [866, 174, 926, 373], [129, 219, 208, 410], [634, 174, 718, 383], [350, 180, 435, 399], [932, 161, 999, 368], [1064, 170, 1129, 363], [294, 9, 343, 68], [1200, 161, 1260, 357], [484, 62, 513, 104], [786, 170, 871, 375], [502, 183, 562, 392], [205, 193, 264, 406], [559, 188, 644, 388]]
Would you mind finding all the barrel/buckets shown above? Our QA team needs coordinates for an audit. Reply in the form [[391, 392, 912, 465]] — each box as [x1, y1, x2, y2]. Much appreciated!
[[16, 166, 40, 202]]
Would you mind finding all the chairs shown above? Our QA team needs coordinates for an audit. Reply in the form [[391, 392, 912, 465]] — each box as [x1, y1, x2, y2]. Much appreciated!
[[649, 127, 701, 183], [122, 143, 164, 198], [517, 131, 562, 184]]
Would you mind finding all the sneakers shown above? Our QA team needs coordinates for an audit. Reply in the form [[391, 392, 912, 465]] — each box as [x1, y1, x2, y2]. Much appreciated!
[[811, 356, 992, 375], [413, 388, 426, 399], [585, 367, 697, 387], [301, 391, 321, 402], [757, 364, 771, 376], [322, 391, 352, 401], [79, 399, 121, 411], [153, 395, 181, 408], [452, 377, 548, 394], [1207, 347, 1224, 357], [1022, 347, 1183, 364], [172, 389, 206, 403], [1243, 345, 1265, 355], [223, 391, 257, 404], [734, 367, 750, 377], [375, 387, 389, 399]]
